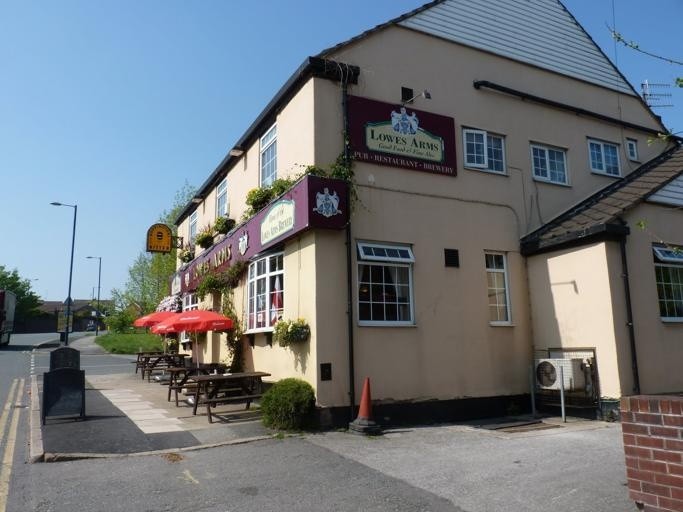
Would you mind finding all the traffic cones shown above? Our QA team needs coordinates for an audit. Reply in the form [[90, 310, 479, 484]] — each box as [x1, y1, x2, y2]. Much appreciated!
[[348, 377, 382, 434]]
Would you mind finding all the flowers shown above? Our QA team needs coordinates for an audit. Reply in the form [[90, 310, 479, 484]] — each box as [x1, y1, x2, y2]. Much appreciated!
[[272, 317, 310, 346]]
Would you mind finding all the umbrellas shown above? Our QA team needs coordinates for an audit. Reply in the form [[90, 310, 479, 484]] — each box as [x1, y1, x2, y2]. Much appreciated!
[[134, 311, 186, 368], [150, 309, 233, 367]]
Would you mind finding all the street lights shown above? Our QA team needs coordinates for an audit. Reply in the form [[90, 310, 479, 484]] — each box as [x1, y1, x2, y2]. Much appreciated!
[[49, 202, 78, 346], [86, 256, 101, 336]]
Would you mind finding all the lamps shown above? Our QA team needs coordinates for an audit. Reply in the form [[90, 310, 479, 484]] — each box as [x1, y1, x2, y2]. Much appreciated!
[[230, 145, 247, 159], [406, 89, 432, 103], [191, 195, 205, 205]]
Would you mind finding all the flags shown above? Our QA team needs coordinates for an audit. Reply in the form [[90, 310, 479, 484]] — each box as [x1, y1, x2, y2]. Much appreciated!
[[269, 264, 281, 326]]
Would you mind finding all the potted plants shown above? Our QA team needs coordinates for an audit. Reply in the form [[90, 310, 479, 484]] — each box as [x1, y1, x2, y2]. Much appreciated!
[[259, 377, 316, 433], [178, 241, 196, 263], [214, 216, 235, 235], [194, 224, 216, 249], [245, 185, 275, 211]]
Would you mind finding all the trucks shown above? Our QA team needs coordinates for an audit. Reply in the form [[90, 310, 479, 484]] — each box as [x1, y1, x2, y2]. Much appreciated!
[[0, 290, 17, 347]]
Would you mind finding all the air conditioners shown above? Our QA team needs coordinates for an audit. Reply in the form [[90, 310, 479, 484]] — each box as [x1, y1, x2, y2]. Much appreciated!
[[535, 358, 586, 391]]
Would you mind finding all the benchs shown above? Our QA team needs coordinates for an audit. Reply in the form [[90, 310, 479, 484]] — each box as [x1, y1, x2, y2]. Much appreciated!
[[132, 360, 263, 404]]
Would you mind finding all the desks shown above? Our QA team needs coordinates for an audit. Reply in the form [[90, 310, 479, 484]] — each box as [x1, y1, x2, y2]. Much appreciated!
[[135, 351, 163, 373], [140, 354, 190, 382], [187, 372, 271, 423]]
